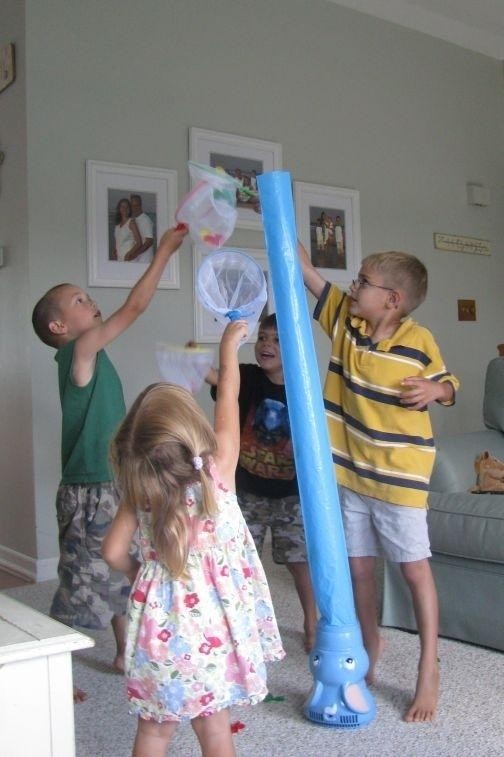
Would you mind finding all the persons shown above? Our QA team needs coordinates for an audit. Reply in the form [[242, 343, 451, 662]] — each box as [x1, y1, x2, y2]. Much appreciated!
[[316, 214, 344, 262], [219, 166, 258, 202], [252, 203, 459, 724], [102, 318, 286, 757], [127, 194, 155, 266], [193, 314, 320, 653], [114, 198, 142, 263], [33, 221, 190, 705]]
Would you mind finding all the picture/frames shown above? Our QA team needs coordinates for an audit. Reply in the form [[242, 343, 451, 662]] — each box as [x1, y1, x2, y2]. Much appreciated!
[[183, 123, 283, 233], [291, 177, 364, 291], [83, 159, 182, 291], [190, 238, 276, 352]]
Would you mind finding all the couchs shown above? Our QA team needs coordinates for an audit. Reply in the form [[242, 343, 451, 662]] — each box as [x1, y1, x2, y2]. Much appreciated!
[[374, 352, 502, 650]]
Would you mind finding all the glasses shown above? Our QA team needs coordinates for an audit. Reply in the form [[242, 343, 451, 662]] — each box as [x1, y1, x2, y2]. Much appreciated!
[[352, 280, 394, 292]]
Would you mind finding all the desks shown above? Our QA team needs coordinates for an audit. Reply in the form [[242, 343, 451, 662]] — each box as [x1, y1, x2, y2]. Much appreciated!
[[0, 587, 97, 755]]
